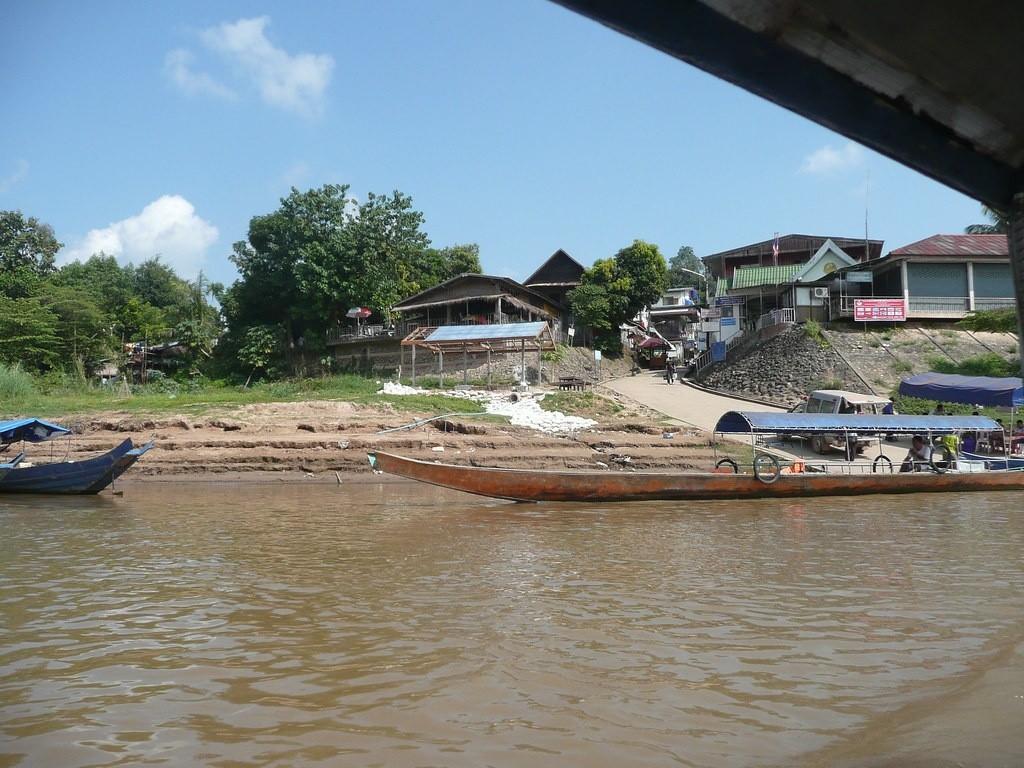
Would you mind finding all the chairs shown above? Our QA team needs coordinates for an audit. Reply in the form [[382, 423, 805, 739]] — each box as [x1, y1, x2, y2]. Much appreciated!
[[975, 431, 989, 454]]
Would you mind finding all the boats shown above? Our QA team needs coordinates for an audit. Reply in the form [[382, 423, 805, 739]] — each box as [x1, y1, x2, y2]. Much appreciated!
[[0, 417, 155, 495], [363, 410, 1024, 503]]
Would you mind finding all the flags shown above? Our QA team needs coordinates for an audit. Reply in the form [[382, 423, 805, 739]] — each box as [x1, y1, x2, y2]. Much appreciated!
[[773, 240, 779, 256]]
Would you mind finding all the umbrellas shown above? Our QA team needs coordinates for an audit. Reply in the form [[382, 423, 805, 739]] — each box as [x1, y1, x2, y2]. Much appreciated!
[[346, 307, 372, 327], [637, 337, 664, 348]]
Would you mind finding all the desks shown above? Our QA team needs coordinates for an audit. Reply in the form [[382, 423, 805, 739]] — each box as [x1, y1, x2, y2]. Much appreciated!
[[997, 433, 1024, 437], [559, 377, 583, 391]]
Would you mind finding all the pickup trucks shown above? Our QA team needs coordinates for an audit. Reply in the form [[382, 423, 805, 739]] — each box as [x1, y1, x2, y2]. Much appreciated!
[[777, 402, 883, 454]]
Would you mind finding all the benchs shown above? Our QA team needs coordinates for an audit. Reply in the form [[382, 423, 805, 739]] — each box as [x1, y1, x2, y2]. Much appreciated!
[[554, 381, 591, 391]]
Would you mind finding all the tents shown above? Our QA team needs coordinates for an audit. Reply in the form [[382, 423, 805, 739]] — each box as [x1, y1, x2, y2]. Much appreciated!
[[899, 372, 1024, 455]]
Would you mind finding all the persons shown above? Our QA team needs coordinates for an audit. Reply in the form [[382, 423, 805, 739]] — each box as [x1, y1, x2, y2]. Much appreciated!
[[1011, 420, 1024, 454], [770, 307, 778, 318], [989, 418, 1006, 454], [882, 397, 895, 441], [666, 357, 675, 385], [966, 412, 980, 442], [928, 404, 944, 415], [901, 436, 931, 471]]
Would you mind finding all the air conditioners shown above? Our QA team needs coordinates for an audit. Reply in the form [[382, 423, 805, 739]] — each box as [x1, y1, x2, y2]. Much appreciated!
[[813, 287, 828, 298]]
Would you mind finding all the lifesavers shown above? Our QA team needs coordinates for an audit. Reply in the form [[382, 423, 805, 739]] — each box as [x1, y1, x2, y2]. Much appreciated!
[[930, 445, 951, 475], [873, 455, 893, 474], [714, 458, 738, 475], [753, 452, 780, 485]]
